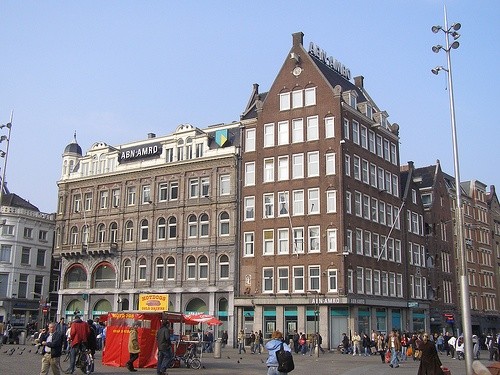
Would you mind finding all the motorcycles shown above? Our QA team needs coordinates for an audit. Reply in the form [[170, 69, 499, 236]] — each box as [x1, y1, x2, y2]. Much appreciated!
[[455, 333, 481, 360]]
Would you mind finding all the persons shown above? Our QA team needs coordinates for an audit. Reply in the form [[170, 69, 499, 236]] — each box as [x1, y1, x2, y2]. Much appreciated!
[[126, 323, 140, 372], [156, 321, 172, 375], [197, 325, 499, 375], [0, 316, 105, 375], [264, 330, 292, 375]]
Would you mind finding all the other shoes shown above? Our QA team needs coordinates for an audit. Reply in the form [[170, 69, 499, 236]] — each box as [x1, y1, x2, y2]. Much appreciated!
[[389, 364, 393, 368], [126, 362, 137, 372], [359, 354, 362, 356], [251, 352, 255, 354], [160, 368, 168, 375], [69, 369, 74, 374], [238, 352, 241, 354], [395, 365, 399, 368], [258, 352, 261, 354], [353, 353, 356, 355], [244, 350, 246, 353]]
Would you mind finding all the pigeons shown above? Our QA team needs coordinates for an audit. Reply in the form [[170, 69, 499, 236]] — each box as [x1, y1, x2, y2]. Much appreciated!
[[226, 355, 264, 363], [3, 347, 38, 355]]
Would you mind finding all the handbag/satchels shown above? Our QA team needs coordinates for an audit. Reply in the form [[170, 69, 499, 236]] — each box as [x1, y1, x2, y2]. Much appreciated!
[[385, 351, 391, 363], [298, 338, 306, 346], [276, 348, 294, 372]]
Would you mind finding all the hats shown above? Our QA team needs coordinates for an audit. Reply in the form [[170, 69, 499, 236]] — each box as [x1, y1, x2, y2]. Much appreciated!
[[132, 323, 138, 327]]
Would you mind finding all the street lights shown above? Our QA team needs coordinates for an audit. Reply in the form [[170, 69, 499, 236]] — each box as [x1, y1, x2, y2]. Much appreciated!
[[431, 5, 475, 375], [0, 108, 14, 207]]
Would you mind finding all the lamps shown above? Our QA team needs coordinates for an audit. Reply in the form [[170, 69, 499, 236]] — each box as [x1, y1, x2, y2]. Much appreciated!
[[205, 195, 209, 199], [75, 211, 78, 213], [113, 206, 118, 208], [148, 201, 155, 205]]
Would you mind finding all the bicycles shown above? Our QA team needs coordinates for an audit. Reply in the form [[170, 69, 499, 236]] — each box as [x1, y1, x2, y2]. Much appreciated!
[[169, 344, 201, 370], [58, 337, 94, 375]]
